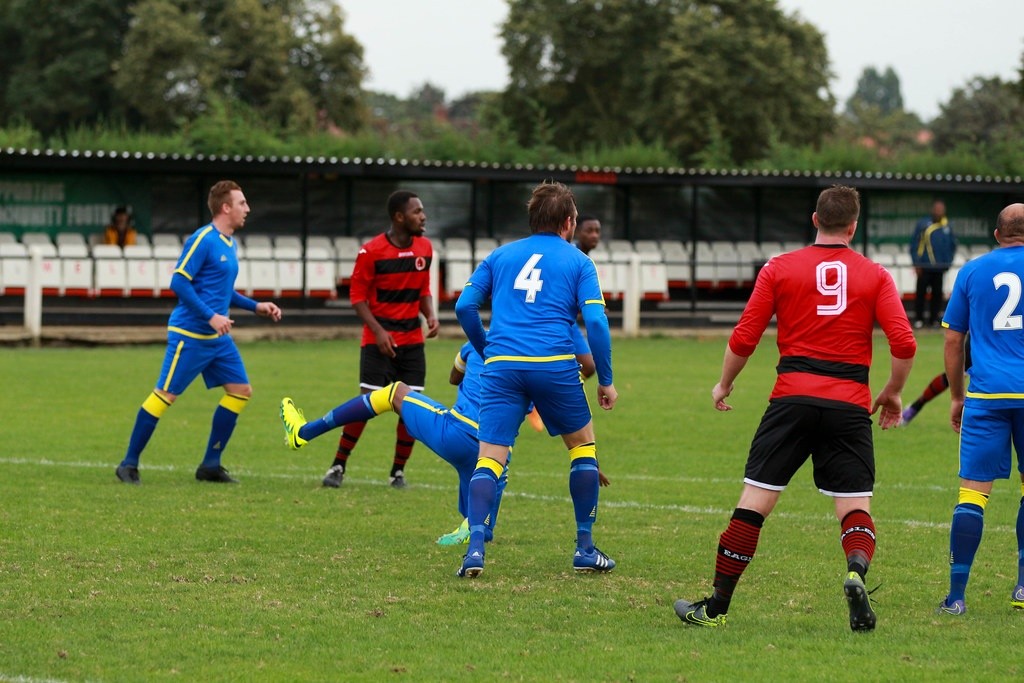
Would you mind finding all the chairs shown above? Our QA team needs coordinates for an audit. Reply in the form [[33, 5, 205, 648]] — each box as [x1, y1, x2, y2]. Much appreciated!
[[0, 229, 1000, 311]]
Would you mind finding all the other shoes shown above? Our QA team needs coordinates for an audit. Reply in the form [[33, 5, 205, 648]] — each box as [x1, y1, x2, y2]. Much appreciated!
[[913, 320, 924, 329], [929, 321, 940, 328]]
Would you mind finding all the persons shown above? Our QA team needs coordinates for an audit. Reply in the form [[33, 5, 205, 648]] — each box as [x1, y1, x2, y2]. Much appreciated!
[[910, 202, 957, 328], [113, 180, 281, 483], [104, 210, 137, 247], [323, 191, 437, 490], [893, 334, 972, 425], [674, 186, 920, 634], [529, 212, 602, 431], [940, 203, 1024, 614], [279, 317, 534, 545], [453, 183, 618, 579]]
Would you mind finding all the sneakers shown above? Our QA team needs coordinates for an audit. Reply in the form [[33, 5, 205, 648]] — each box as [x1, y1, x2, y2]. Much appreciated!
[[936, 594, 966, 618], [456, 548, 486, 579], [1010, 584, 1024, 611], [573, 539, 616, 574], [434, 517, 471, 546], [843, 571, 884, 633], [114, 460, 142, 485], [322, 465, 344, 489], [195, 461, 240, 483], [278, 397, 309, 452], [673, 596, 728, 629], [386, 470, 408, 489]]
